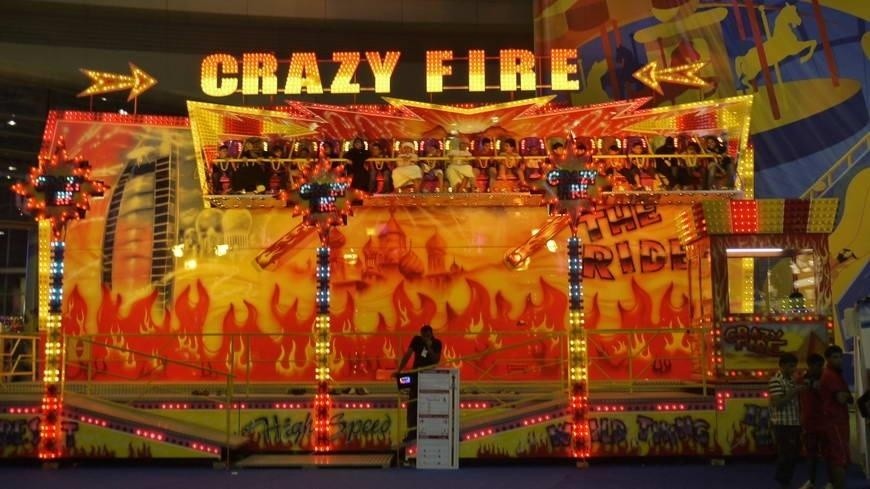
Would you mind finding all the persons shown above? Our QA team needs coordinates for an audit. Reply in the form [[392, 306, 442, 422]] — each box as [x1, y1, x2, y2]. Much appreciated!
[[767, 354, 809, 488], [392, 325, 443, 438], [213, 134, 734, 191], [821, 345, 854, 488], [799, 354, 825, 488], [857, 389, 870, 421]]
